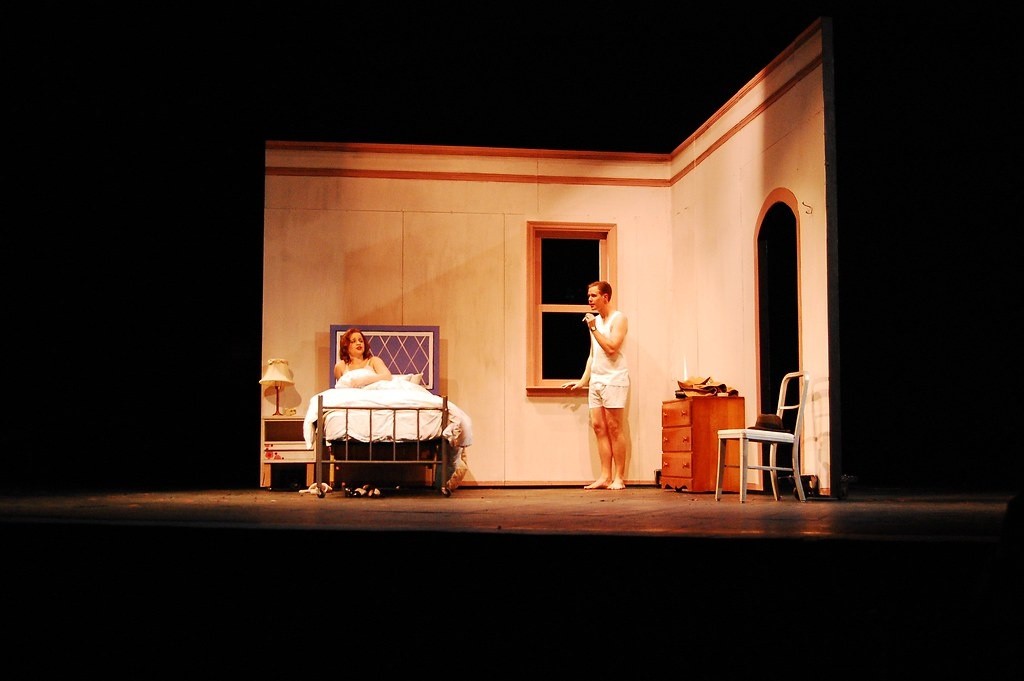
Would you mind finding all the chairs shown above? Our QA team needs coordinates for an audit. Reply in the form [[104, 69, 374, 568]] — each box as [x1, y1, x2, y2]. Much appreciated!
[[714, 372, 809, 504]]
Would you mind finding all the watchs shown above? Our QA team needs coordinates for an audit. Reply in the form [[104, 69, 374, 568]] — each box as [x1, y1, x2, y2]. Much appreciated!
[[590, 326, 597, 331]]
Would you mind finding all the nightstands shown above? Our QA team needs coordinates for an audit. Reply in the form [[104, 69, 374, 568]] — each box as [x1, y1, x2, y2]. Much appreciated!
[[261, 416, 316, 487]]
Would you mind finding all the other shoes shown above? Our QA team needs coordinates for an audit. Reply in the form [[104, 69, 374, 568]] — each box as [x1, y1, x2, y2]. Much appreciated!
[[343, 484, 379, 498]]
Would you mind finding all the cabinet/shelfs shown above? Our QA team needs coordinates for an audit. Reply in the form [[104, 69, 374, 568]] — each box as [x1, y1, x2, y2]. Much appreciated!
[[660, 397, 744, 493]]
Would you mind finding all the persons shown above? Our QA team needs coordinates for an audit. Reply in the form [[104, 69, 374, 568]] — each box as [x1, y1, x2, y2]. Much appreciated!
[[333, 328, 392, 388], [563, 281, 630, 490]]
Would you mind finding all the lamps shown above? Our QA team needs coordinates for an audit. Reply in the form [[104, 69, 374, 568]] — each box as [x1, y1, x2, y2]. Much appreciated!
[[259, 359, 294, 415]]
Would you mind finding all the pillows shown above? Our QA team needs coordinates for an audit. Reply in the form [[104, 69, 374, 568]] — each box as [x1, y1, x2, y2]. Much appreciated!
[[391, 373, 423, 385]]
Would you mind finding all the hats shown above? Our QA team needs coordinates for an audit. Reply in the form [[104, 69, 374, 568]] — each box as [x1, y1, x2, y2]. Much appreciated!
[[747, 414, 790, 432]]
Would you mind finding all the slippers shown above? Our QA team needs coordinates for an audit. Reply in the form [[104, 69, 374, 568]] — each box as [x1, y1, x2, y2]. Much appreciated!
[[298, 483, 333, 494]]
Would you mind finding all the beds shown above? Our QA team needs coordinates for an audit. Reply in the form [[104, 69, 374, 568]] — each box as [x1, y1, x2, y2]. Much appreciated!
[[316, 325, 449, 497]]
